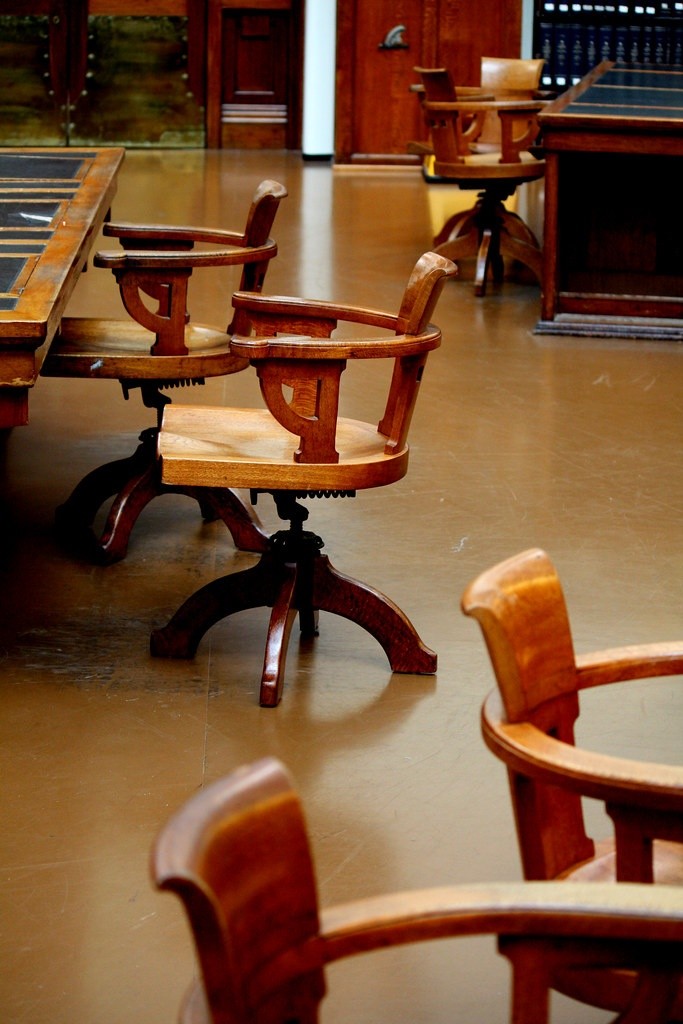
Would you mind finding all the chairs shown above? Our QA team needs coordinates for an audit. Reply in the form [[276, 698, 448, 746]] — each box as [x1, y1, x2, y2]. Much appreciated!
[[458, 544, 683, 1024], [146, 753, 683, 1024], [36, 176, 289, 565], [144, 248, 461, 707], [411, 64, 548, 297], [408, 55, 557, 155]]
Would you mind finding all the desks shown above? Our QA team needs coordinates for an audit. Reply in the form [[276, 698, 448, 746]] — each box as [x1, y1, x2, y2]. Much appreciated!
[[0, 143, 127, 459]]
